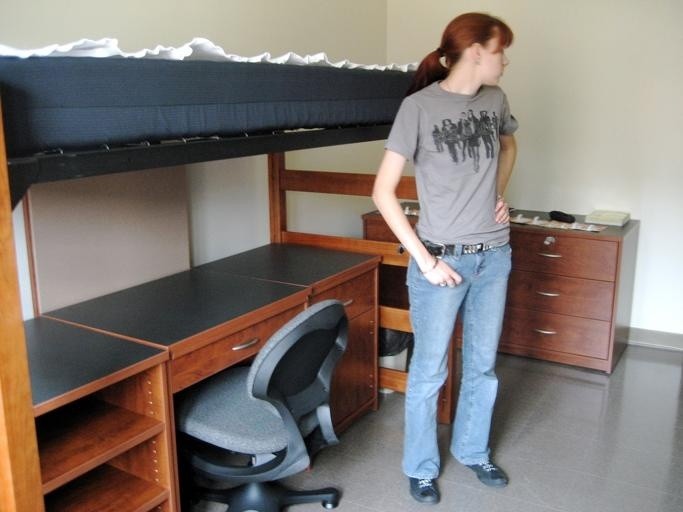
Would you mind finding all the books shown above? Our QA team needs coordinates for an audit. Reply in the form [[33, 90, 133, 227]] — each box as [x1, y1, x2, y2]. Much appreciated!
[[582, 209, 630, 227]]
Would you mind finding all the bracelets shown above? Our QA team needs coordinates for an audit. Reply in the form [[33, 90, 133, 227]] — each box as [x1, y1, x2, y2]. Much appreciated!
[[421, 253, 438, 276]]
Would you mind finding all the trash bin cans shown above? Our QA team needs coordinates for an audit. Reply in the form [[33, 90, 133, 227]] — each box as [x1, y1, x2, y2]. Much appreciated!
[[377, 328, 414, 394]]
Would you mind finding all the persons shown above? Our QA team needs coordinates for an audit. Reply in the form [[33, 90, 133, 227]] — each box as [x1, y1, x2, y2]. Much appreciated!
[[366, 10, 521, 507], [429, 107, 500, 173]]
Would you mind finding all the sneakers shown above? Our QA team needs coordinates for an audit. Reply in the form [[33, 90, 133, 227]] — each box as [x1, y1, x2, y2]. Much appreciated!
[[466, 460, 509, 487], [408, 476, 440, 505]]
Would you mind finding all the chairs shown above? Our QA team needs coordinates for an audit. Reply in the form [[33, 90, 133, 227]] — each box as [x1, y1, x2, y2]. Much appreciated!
[[176, 298, 351, 510]]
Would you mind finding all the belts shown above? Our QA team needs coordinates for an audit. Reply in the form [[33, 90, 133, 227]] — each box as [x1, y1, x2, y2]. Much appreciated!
[[422, 242, 493, 256]]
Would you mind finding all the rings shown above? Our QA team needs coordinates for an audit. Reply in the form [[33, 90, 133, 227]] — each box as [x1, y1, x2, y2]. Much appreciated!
[[439, 282, 448, 287]]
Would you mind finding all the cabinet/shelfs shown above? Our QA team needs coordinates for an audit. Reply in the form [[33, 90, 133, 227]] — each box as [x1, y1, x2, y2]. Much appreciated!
[[360, 202, 640, 376], [22, 241, 383, 511]]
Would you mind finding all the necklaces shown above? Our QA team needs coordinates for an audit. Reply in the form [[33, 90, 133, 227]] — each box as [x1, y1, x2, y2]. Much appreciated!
[[444, 77, 452, 92]]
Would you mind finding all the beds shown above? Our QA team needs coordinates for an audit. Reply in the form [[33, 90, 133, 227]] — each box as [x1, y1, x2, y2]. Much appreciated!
[[0, 49, 438, 187]]
